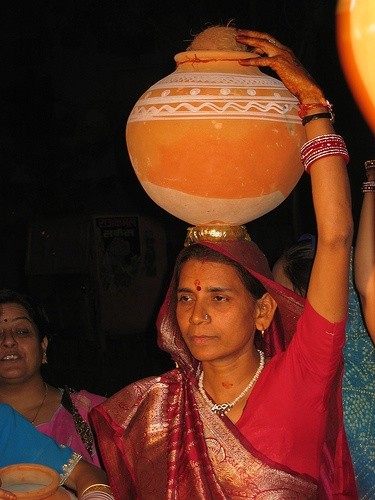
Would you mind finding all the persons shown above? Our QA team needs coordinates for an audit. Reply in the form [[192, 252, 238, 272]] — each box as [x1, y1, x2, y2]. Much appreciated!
[[271, 156, 375, 500], [88, 29, 358, 500], [0, 403, 115, 500], [0, 290, 108, 472]]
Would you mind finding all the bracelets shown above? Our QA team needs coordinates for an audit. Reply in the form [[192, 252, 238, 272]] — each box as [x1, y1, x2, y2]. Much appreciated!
[[79, 483, 115, 500], [300, 133, 350, 173], [364, 159, 375, 170], [298, 100, 336, 125], [361, 181, 375, 193]]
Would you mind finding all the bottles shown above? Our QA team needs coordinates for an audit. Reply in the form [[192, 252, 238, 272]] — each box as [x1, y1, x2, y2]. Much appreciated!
[[126, 50, 305, 225], [0, 463, 71, 500]]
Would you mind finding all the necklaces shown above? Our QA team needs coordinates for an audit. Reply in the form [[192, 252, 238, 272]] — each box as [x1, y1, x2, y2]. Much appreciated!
[[31, 383, 48, 425], [196, 349, 265, 416]]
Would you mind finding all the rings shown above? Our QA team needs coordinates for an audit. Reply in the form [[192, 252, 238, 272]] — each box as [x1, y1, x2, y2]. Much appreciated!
[[268, 38, 279, 45]]
